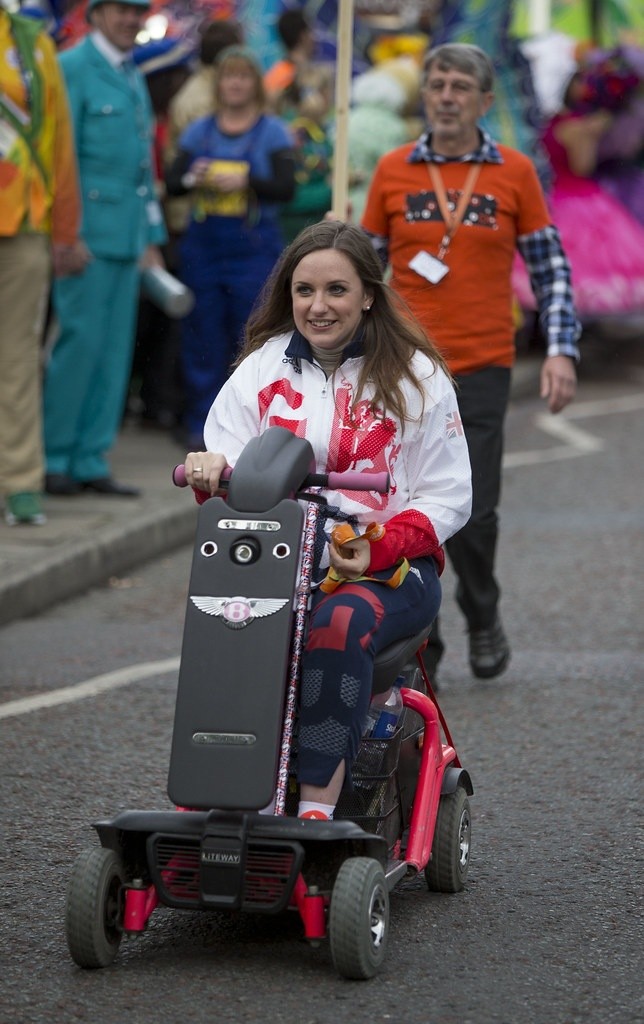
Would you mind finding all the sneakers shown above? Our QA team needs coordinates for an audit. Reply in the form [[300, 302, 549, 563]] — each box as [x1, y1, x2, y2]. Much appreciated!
[[461, 620, 511, 680]]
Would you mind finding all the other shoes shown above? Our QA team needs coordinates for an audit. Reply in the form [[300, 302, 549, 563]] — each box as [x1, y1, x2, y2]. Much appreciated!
[[49, 474, 138, 499], [5, 493, 46, 526]]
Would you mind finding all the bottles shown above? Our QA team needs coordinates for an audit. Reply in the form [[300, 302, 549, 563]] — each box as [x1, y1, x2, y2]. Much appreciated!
[[349, 675, 406, 792]]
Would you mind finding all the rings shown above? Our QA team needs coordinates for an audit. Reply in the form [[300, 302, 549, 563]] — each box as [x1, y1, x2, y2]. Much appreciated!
[[193, 467, 204, 473]]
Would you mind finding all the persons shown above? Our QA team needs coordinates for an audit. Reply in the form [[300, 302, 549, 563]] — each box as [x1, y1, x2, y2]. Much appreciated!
[[183, 218, 475, 819], [355, 42, 583, 698], [1, 0, 643, 525]]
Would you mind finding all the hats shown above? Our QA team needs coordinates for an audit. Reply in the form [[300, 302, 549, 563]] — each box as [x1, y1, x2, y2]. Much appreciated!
[[86, 0, 149, 25]]
